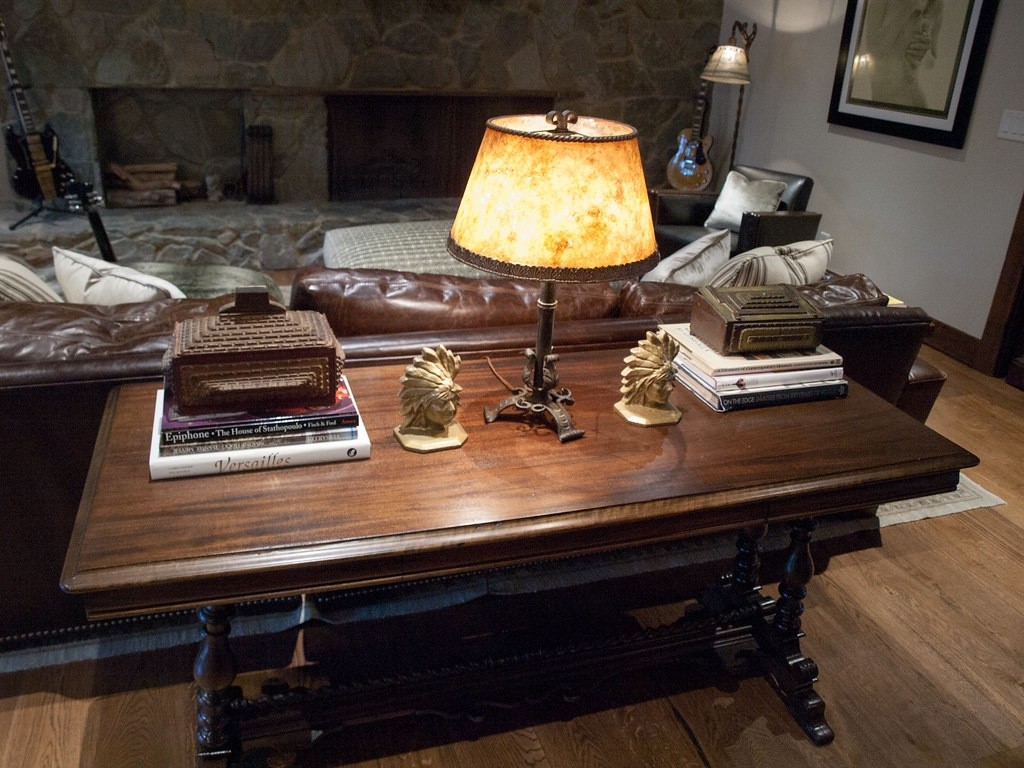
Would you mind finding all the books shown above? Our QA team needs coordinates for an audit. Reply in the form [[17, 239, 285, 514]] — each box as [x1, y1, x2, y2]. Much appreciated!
[[149, 375, 371, 481], [656, 324, 849, 412]]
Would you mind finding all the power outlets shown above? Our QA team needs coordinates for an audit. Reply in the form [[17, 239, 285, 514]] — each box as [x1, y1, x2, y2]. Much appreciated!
[[818, 232, 831, 241]]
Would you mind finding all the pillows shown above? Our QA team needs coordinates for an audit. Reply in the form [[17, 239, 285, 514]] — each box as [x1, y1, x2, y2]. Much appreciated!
[[50, 245, 187, 307], [703, 170, 788, 233], [640, 228, 732, 287], [0, 255, 65, 303], [703, 239, 835, 288]]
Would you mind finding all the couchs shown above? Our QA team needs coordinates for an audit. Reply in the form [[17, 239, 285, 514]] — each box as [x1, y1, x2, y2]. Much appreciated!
[[0, 234, 949, 657], [648, 164, 822, 260]]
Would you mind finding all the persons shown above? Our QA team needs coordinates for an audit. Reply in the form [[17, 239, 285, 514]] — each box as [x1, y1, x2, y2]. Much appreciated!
[[866, 0, 944, 109], [397, 345, 463, 431], [621, 332, 678, 407]]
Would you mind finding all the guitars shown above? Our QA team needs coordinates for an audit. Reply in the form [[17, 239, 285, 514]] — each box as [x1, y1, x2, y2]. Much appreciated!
[[0, 22, 74, 201], [666, 44, 720, 191]]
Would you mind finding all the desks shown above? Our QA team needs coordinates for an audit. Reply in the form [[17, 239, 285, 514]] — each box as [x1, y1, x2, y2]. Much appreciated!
[[59, 350, 981, 768]]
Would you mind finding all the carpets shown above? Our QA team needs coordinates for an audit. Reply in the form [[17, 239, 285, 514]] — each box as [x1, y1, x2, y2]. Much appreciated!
[[0, 471, 1008, 673]]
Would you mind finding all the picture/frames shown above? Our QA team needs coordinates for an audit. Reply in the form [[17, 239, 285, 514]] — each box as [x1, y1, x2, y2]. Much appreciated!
[[826, 0, 1001, 151]]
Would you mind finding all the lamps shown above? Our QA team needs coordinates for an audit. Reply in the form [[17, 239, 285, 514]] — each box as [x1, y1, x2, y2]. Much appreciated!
[[446, 111, 661, 443], [699, 20, 757, 172]]
